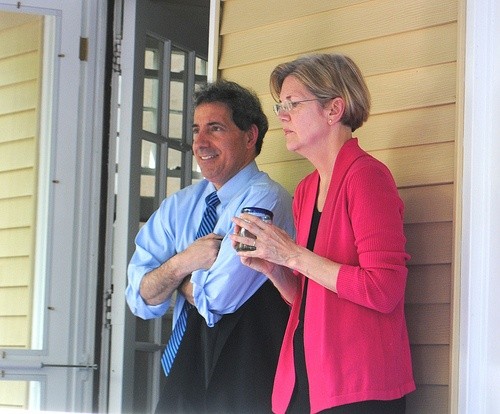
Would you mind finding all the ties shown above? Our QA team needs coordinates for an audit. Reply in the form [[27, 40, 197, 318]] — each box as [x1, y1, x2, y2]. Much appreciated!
[[160, 190, 221, 378]]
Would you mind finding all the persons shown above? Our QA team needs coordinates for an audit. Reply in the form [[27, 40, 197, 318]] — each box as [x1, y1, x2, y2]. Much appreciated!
[[122, 78, 300, 414], [226, 53, 418, 414]]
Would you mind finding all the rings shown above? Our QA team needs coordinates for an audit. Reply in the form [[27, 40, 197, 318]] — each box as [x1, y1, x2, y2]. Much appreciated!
[[253, 239, 256, 247]]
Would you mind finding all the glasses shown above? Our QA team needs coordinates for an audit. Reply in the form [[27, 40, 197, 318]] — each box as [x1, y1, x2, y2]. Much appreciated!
[[272, 98, 318, 115]]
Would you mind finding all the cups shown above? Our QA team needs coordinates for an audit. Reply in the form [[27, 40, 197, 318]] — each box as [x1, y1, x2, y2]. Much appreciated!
[[234, 207, 273, 251]]
